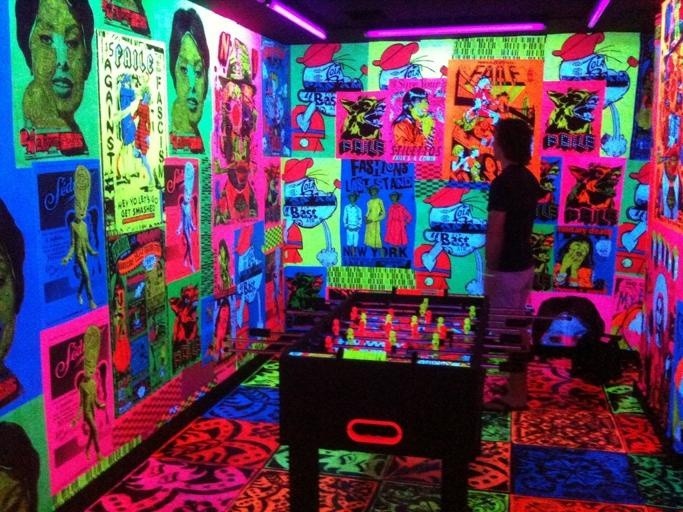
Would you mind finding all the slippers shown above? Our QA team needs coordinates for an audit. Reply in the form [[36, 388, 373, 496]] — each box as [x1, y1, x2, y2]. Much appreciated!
[[484, 396, 528, 412]]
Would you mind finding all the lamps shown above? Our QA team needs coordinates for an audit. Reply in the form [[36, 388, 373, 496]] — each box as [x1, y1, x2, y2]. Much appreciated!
[[266, 1, 329, 41]]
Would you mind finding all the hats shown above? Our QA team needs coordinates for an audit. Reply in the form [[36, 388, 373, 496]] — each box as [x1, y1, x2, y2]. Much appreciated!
[[220, 61, 257, 96]]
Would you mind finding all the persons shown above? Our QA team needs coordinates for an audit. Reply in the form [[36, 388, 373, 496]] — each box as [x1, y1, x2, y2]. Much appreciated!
[[71, 359, 106, 461], [177, 194, 197, 274], [63, 201, 100, 309], [0, 420, 39, 510], [133, 91, 153, 178], [0, 203, 26, 406], [15, 0, 94, 153], [481, 118, 541, 413], [344, 184, 411, 257], [168, 9, 210, 152], [393, 88, 435, 160]]
